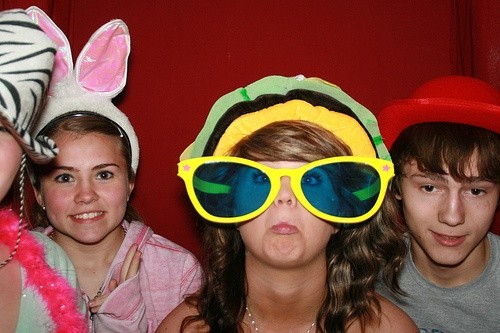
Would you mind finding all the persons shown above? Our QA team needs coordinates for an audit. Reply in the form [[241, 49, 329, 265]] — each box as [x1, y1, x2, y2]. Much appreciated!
[[375, 75, 500, 333], [154, 74, 421, 333], [0, 8, 91, 333], [24, 6, 206, 333]]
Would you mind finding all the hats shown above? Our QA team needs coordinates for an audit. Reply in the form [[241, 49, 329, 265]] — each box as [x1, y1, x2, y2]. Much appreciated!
[[22, 7, 139, 175], [0, 8, 59, 165], [378, 76, 500, 152], [180, 74, 393, 161]]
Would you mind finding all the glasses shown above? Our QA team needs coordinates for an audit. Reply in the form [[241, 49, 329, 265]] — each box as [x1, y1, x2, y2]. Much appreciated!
[[179, 155, 395, 223]]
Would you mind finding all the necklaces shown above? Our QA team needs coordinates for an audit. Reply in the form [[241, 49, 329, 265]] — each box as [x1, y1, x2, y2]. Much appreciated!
[[246, 304, 315, 333], [49, 228, 128, 298]]
